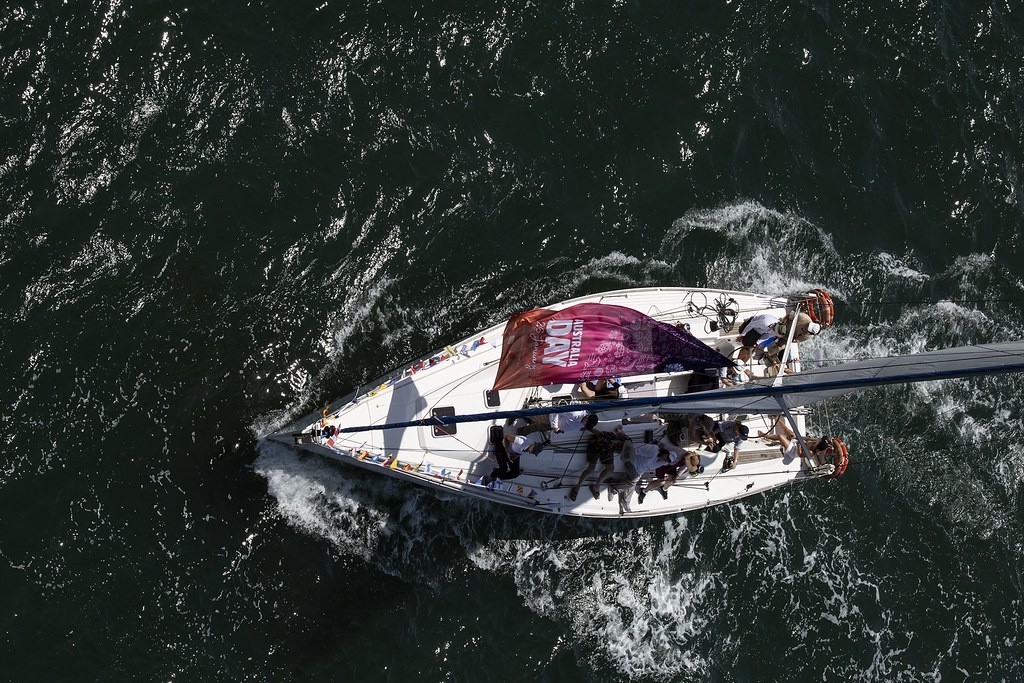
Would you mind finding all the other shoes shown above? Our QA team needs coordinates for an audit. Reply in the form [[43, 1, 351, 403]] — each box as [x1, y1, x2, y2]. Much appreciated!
[[660, 485, 667, 499], [639, 488, 646, 504], [507, 418, 513, 426], [490, 468, 497, 481]]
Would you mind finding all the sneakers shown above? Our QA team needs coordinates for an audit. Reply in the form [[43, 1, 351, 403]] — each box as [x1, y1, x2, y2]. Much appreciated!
[[608, 485, 617, 501], [620, 498, 630, 511], [758, 352, 768, 365]]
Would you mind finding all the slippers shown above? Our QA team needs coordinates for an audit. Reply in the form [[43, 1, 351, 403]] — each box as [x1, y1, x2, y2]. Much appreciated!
[[590, 485, 600, 500], [570, 487, 578, 501]]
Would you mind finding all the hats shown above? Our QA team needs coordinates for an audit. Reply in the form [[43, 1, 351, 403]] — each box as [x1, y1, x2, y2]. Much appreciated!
[[808, 323, 820, 334], [733, 359, 744, 374], [669, 451, 677, 464], [702, 414, 714, 432], [534, 441, 544, 457], [819, 436, 828, 451], [621, 440, 634, 462], [618, 386, 628, 398], [586, 415, 598, 430], [739, 425, 749, 441], [684, 451, 700, 472], [775, 322, 790, 338]]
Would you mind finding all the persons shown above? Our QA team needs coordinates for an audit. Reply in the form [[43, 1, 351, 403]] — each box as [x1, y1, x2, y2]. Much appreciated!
[[489, 312, 829, 504]]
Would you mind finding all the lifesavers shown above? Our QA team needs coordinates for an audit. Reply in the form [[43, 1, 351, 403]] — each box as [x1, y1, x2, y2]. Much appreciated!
[[814, 436, 850, 480], [801, 287, 834, 330]]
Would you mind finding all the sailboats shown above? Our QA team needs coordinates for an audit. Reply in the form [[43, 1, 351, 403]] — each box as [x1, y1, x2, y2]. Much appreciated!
[[263, 283, 1024, 526]]
